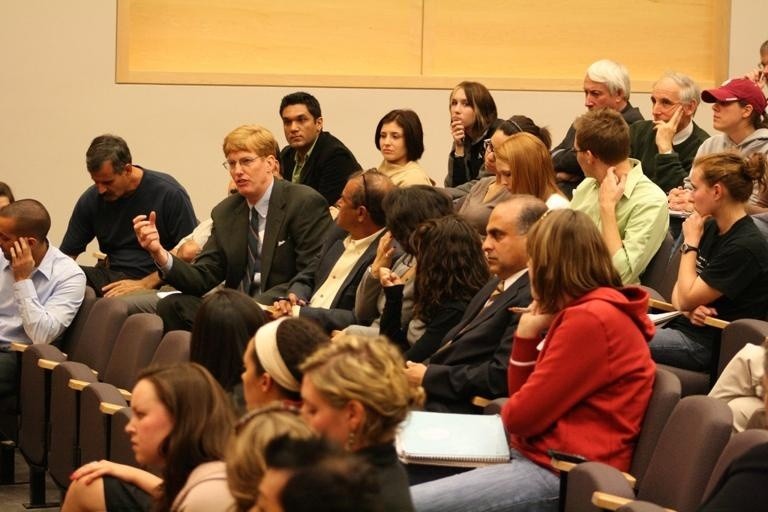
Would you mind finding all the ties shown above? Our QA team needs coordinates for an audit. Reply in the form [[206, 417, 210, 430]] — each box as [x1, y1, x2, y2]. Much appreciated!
[[480, 278, 505, 314], [237, 206, 259, 294]]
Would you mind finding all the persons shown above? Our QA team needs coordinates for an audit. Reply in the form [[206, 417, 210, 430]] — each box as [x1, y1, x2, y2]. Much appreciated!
[[449, 115, 551, 233], [432, 81, 508, 188], [750, 42, 768, 127], [103, 179, 239, 314], [62, 135, 200, 298], [406, 207, 657, 510], [132, 125, 333, 331], [275, 92, 364, 206], [564, 106, 669, 297], [126, 362, 238, 511], [355, 185, 453, 337], [629, 73, 711, 241], [299, 334, 427, 512], [240, 317, 330, 412], [1, 183, 14, 211], [225, 402, 323, 512], [640, 150, 768, 371], [1, 199, 88, 482], [381, 216, 491, 361], [495, 132, 571, 209], [325, 109, 433, 218], [247, 430, 384, 512], [59, 289, 269, 511], [668, 75, 768, 214], [268, 169, 401, 331], [550, 59, 644, 199], [402, 196, 547, 413]]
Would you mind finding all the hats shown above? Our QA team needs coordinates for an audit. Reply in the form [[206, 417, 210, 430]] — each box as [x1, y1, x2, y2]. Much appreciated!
[[701, 76, 767, 114]]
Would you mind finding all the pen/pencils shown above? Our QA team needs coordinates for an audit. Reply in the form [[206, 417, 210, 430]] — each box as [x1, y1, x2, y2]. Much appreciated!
[[508, 307, 531, 313], [272, 295, 311, 304]]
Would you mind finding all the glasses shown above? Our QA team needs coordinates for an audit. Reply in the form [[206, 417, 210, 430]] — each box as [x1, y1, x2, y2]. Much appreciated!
[[222, 154, 264, 172], [360, 167, 377, 210], [483, 138, 496, 153], [569, 146, 598, 159], [757, 62, 768, 70]]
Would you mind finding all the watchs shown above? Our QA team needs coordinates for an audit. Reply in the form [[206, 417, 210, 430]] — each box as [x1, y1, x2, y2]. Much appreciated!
[[678, 242, 701, 252]]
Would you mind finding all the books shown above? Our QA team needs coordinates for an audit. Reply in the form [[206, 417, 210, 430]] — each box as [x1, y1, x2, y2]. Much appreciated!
[[645, 310, 681, 327], [395, 410, 512, 469]]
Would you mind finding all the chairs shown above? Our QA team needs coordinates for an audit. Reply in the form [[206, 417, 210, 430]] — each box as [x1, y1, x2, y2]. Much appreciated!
[[68, 329, 191, 464], [647, 298, 730, 396], [546, 395, 734, 512], [111, 408, 140, 468], [640, 245, 682, 302], [9, 297, 129, 509], [640, 232, 674, 289], [38, 312, 164, 489], [59, 285, 97, 354], [472, 395, 507, 414], [628, 370, 682, 489], [717, 319, 768, 378]]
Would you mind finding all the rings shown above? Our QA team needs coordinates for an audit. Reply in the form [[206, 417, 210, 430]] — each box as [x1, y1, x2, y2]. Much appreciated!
[[385, 253, 391, 258]]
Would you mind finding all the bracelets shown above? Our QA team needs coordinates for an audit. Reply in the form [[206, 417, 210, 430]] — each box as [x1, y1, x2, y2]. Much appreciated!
[[366, 265, 378, 280]]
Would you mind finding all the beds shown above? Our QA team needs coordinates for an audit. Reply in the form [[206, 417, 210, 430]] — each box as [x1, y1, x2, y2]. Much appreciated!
[[615, 427, 767, 512]]
